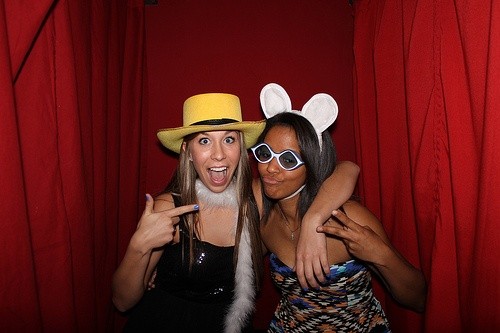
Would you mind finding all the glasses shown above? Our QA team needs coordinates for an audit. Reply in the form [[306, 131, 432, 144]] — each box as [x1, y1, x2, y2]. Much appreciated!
[[249, 143, 305, 171]]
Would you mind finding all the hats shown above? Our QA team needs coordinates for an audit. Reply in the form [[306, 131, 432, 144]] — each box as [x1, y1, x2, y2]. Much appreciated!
[[156, 93, 267, 154]]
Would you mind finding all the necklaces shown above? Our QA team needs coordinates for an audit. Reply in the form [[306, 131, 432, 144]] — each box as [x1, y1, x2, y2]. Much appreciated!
[[275, 204, 301, 241]]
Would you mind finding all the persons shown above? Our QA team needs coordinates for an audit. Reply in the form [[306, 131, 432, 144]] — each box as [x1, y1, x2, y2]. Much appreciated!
[[147, 82, 426, 332], [111, 92, 360, 333]]
